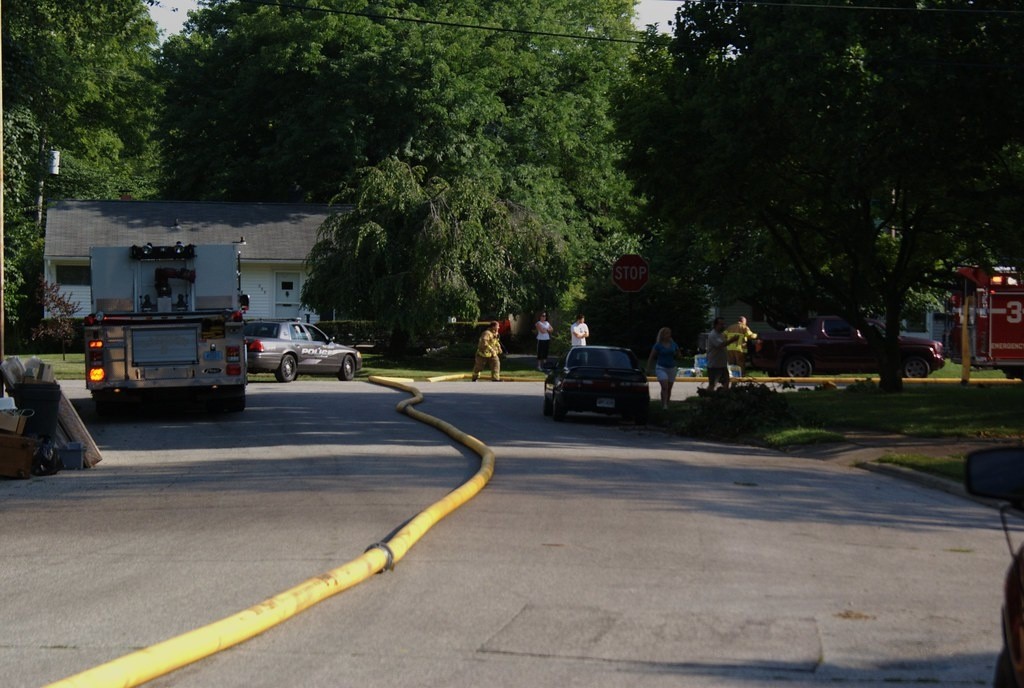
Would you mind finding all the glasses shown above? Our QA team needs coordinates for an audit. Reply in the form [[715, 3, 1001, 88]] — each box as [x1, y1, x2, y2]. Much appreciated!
[[541, 315, 546, 317]]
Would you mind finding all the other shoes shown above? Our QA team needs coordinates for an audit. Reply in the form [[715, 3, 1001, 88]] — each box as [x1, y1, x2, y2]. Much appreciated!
[[492, 379, 504, 382], [471, 379, 477, 382], [537, 367, 542, 371], [663, 405, 668, 410]]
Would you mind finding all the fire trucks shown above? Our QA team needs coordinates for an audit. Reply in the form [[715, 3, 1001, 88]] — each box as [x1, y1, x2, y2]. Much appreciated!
[[945, 263, 1024, 380], [81, 233, 250, 418]]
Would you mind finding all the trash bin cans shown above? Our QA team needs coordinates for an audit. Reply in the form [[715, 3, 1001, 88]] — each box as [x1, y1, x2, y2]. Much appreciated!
[[16, 381, 62, 442]]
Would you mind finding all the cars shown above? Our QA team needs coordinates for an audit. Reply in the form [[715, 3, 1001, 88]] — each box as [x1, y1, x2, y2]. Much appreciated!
[[749, 314, 945, 379], [246, 317, 363, 383], [542, 344, 649, 426], [964, 445, 1024, 688]]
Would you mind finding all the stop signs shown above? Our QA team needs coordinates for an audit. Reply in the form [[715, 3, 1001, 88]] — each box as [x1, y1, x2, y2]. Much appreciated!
[[611, 253, 650, 292]]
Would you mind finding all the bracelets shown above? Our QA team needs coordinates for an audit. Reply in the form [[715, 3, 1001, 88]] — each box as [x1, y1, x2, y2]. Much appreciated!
[[547, 329, 548, 331]]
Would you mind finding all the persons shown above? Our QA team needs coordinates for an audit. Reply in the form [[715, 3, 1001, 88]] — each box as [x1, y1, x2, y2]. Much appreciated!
[[536, 311, 553, 373], [497, 327, 508, 354], [721, 316, 758, 377], [703, 317, 739, 401], [571, 315, 590, 346], [472, 321, 503, 382]]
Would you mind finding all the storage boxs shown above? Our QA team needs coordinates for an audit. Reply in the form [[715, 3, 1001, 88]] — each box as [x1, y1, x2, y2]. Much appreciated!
[[0, 411, 27, 436]]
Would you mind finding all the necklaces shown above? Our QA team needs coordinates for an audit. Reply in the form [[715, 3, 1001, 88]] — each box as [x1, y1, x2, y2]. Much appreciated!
[[647, 327, 681, 410]]
